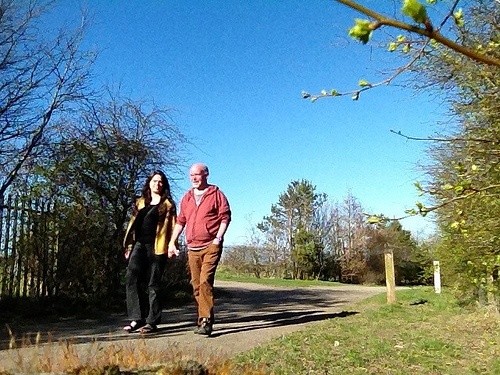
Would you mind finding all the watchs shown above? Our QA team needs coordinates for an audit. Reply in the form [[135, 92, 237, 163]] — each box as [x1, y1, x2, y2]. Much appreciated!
[[215, 236, 223, 241]]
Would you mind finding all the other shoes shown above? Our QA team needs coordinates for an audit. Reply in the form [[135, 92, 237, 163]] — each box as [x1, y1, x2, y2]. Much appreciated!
[[194, 327, 201, 334], [198, 321, 212, 335]]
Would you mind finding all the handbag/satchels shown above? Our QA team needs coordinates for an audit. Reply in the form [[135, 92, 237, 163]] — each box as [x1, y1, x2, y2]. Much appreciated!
[[125, 244, 132, 259]]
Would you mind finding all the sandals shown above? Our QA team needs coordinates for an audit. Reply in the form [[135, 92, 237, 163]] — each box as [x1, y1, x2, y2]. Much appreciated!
[[140, 325, 157, 334], [124, 319, 146, 332]]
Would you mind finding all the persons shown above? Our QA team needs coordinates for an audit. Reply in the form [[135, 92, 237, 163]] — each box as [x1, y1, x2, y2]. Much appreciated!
[[168, 163, 231, 335], [123, 170, 179, 334]]
